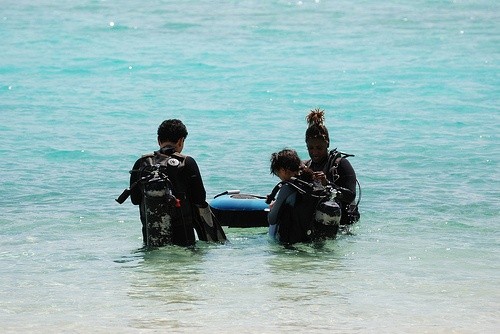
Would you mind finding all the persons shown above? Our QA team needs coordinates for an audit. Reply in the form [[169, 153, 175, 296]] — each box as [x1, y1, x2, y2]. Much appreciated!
[[265, 149, 341, 245], [301, 108, 361, 225], [130, 120, 206, 246]]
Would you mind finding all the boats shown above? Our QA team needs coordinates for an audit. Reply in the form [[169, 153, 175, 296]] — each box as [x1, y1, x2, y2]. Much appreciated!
[[208, 188, 276, 228]]
